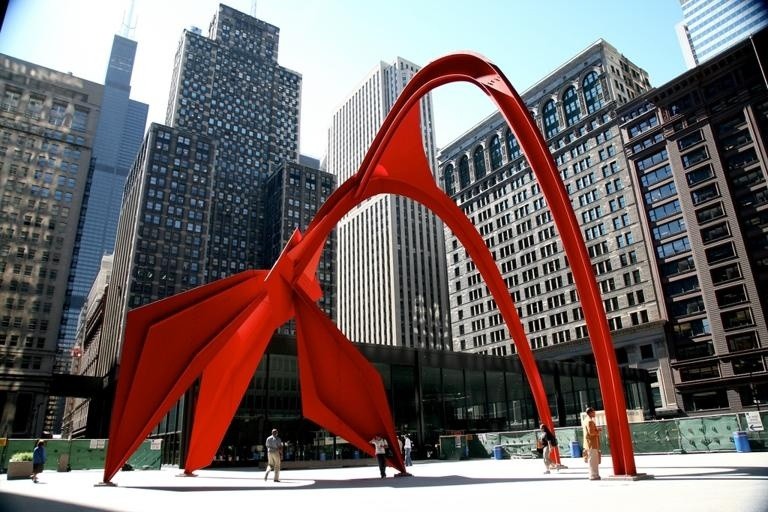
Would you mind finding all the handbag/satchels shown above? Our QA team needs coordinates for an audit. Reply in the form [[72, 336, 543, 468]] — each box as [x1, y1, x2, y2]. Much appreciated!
[[551, 439, 558, 447], [583, 449, 601, 463]]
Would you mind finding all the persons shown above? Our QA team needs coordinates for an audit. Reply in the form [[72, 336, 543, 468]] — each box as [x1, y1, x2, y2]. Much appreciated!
[[396, 435, 403, 454], [30, 439, 45, 483], [369, 432, 389, 479], [582, 407, 603, 480], [539, 423, 561, 474], [264, 428, 283, 481], [403, 433, 413, 466]]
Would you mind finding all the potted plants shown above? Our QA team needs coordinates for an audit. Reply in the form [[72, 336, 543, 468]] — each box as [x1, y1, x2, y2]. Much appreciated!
[[7, 452, 34, 477]]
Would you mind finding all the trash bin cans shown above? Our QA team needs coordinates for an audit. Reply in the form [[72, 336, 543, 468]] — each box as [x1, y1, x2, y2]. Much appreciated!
[[354, 450, 359, 458], [733, 430, 750, 452], [494, 446, 501, 459], [465, 447, 469, 457], [570, 441, 580, 457], [320, 453, 326, 460], [57, 454, 68, 471]]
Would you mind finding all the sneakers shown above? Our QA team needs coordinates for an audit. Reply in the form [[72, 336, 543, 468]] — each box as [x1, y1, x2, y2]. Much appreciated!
[[381, 476, 386, 480], [544, 464, 560, 474], [31, 475, 39, 483], [589, 476, 601, 480], [265, 475, 280, 482]]
